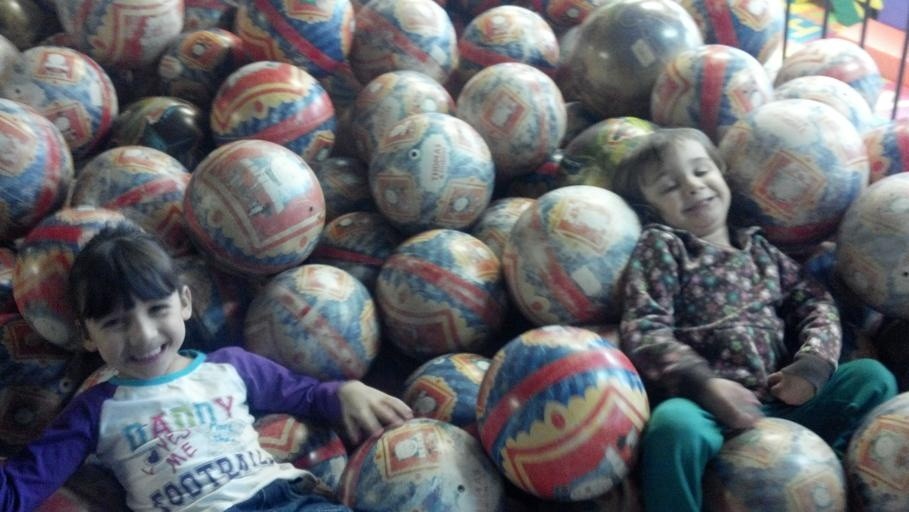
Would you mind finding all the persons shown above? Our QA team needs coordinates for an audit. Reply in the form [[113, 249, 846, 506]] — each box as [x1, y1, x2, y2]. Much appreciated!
[[614, 128, 897, 510], [1, 219, 411, 512]]
